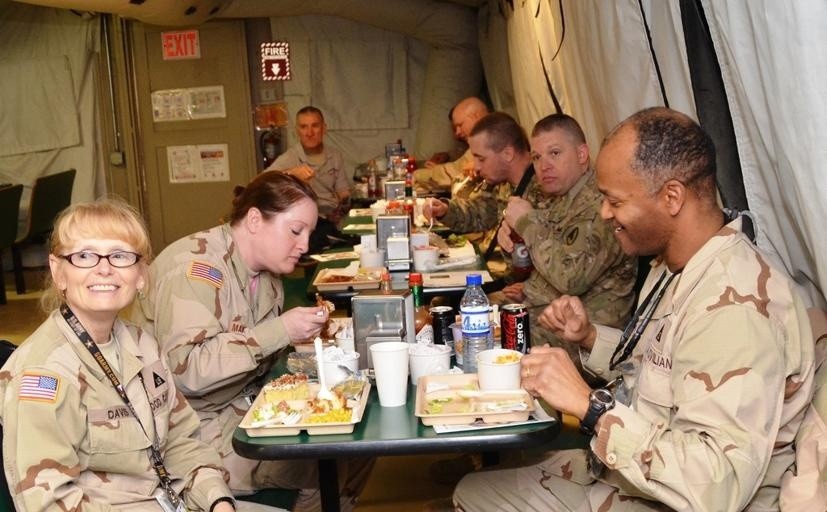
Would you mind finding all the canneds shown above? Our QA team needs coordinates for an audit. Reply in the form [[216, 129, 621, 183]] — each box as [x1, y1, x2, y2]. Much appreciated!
[[429, 305, 457, 368], [500, 303, 530, 354]]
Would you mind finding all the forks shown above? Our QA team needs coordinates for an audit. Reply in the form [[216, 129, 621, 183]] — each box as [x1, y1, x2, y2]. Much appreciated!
[[427, 199, 434, 235], [313, 336, 335, 403], [251, 412, 302, 429]]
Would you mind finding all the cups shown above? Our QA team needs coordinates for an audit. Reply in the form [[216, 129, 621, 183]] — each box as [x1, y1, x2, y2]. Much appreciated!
[[314, 348, 361, 383], [369, 341, 409, 408], [356, 248, 386, 270], [411, 246, 440, 273], [474, 346, 526, 390]]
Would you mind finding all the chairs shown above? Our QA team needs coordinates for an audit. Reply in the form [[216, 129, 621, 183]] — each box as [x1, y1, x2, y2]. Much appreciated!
[[1, 184, 25, 305], [16, 169, 76, 293]]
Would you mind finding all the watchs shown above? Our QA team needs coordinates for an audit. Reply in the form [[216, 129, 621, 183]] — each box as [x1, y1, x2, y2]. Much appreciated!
[[576, 386, 616, 437]]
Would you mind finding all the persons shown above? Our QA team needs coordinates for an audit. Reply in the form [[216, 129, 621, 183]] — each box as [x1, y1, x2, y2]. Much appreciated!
[[428, 114, 641, 486], [1, 200, 291, 512], [451, 106, 816, 512], [262, 105, 356, 278], [412, 96, 548, 283], [116, 170, 377, 512]]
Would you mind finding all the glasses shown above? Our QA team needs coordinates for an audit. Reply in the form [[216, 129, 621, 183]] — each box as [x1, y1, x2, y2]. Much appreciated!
[[59, 251, 142, 268]]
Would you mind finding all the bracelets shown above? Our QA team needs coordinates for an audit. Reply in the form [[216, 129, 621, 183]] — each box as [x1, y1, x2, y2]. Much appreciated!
[[210, 495, 236, 511]]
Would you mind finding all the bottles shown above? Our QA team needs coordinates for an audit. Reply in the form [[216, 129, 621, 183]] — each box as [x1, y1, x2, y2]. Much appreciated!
[[459, 271, 496, 373], [368, 139, 415, 233], [380, 273, 394, 291], [408, 272, 435, 344]]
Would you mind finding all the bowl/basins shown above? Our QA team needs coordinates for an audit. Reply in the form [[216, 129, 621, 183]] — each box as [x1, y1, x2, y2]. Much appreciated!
[[409, 344, 452, 385]]
